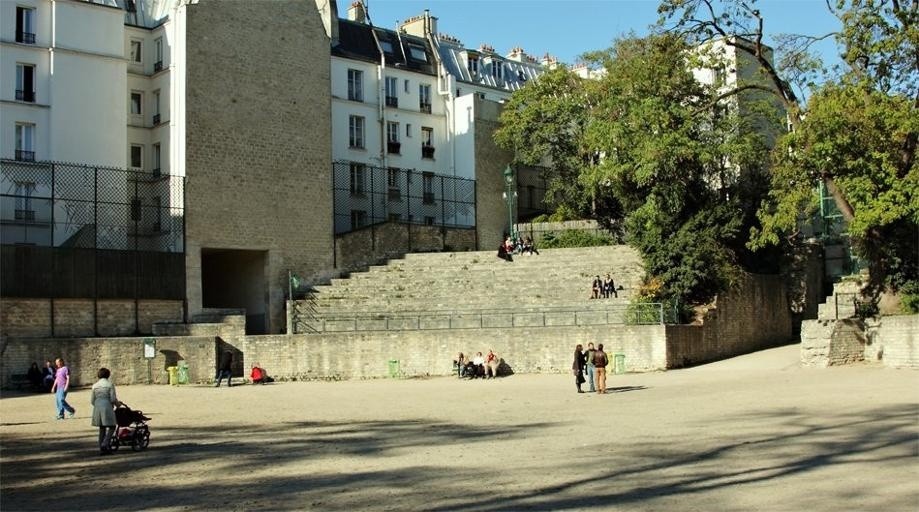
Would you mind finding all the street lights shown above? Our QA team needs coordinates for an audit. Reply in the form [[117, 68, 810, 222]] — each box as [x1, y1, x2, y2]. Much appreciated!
[[503, 166, 517, 242]]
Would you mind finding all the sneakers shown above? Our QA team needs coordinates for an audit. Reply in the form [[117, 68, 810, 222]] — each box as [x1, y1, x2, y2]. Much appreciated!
[[575, 388, 607, 396], [100, 445, 113, 455], [55, 410, 75, 420]]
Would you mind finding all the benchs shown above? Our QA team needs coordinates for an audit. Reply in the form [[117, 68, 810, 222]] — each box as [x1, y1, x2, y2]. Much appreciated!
[[451, 358, 505, 378], [10, 373, 55, 393]]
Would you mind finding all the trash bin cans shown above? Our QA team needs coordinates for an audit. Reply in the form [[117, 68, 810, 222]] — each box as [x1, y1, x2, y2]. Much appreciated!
[[388, 361, 399, 379], [166, 366, 188, 385], [608, 354, 625, 376]]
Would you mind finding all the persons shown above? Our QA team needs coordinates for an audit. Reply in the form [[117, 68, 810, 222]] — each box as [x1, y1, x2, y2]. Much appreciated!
[[51, 357, 75, 420], [472, 351, 484, 379], [588, 275, 602, 299], [484, 349, 498, 380], [583, 342, 597, 392], [251, 366, 264, 384], [498, 234, 540, 262], [90, 368, 122, 455], [28, 362, 42, 392], [590, 344, 608, 394], [215, 345, 233, 387], [42, 360, 56, 392], [456, 352, 474, 378], [602, 273, 614, 299], [572, 344, 587, 393]]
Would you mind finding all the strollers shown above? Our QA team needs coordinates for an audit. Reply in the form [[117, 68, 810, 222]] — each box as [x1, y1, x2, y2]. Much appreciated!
[[109, 402, 151, 452]]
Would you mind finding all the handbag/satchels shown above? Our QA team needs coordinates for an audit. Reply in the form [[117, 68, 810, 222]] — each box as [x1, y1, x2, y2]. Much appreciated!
[[576, 375, 586, 385]]
